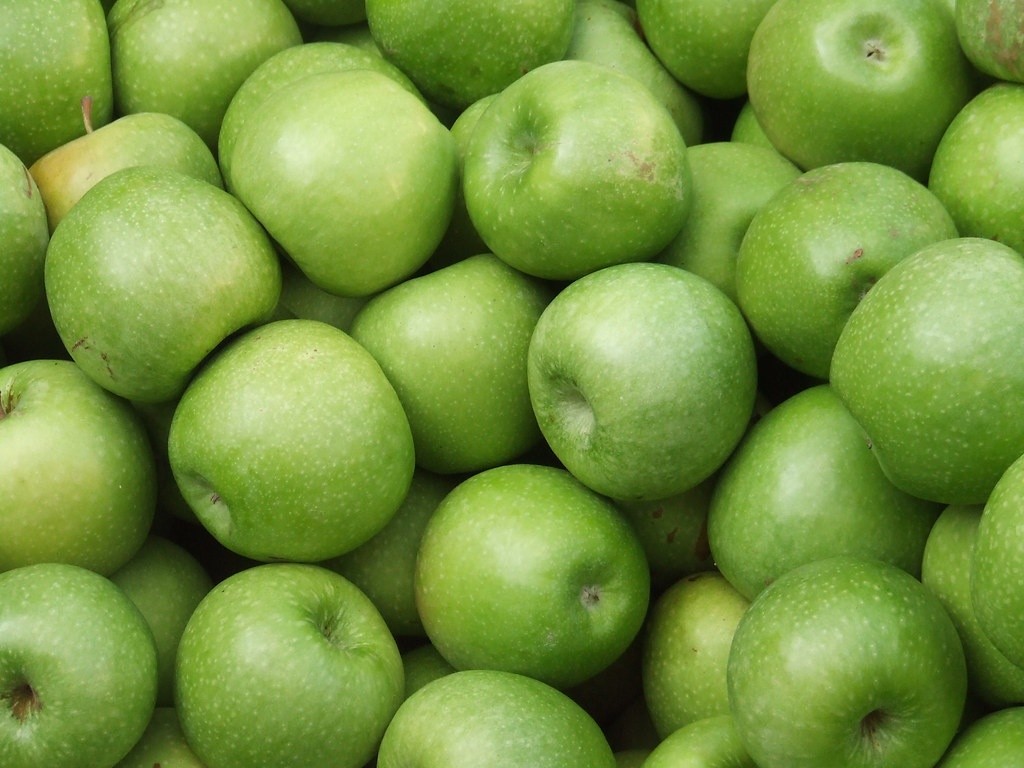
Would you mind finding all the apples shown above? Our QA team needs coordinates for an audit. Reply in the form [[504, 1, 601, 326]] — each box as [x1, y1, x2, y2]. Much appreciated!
[[0, 0, 1024, 768]]
